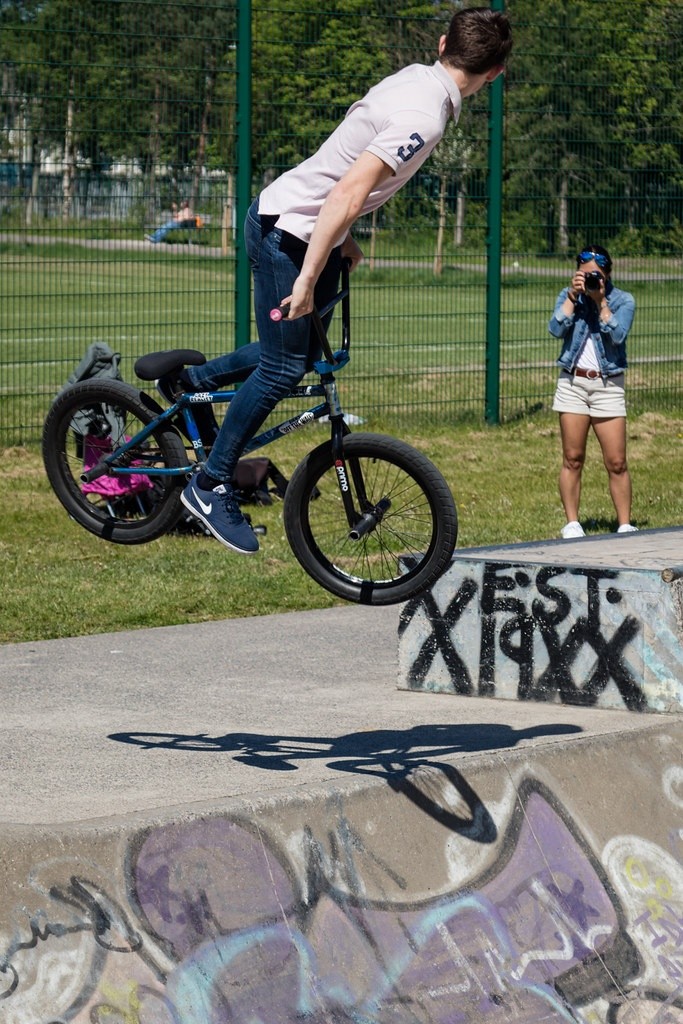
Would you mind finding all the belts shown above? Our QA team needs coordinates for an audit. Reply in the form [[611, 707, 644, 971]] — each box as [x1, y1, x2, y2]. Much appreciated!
[[564, 365, 603, 381]]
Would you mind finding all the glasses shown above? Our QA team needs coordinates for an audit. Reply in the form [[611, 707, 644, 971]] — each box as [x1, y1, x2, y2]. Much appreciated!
[[577, 252, 611, 268]]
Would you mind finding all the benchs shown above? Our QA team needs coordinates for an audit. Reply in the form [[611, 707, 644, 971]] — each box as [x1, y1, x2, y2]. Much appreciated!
[[156, 210, 212, 245]]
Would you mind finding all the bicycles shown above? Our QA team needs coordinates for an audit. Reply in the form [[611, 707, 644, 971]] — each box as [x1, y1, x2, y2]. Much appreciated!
[[41, 251, 460, 607]]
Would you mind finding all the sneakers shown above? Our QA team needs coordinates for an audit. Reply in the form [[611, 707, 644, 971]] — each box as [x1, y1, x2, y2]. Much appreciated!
[[179, 472, 260, 554], [160, 376, 221, 446]]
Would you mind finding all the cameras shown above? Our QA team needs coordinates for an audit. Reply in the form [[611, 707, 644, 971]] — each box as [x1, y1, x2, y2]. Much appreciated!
[[583, 270, 604, 292]]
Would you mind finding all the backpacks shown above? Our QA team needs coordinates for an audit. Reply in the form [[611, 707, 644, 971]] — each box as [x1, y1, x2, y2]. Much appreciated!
[[52, 342, 126, 448]]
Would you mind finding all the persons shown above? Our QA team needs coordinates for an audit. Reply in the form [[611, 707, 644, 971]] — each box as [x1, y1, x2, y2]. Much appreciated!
[[144, 200, 188, 244], [154, 6, 514, 554], [549, 245, 638, 539]]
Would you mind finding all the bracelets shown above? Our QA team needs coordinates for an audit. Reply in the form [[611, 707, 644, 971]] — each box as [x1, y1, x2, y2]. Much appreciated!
[[568, 291, 576, 304], [597, 303, 608, 310]]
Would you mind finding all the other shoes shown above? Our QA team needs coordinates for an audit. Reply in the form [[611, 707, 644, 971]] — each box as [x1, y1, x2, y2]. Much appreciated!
[[144, 233, 157, 243], [617, 525, 636, 535], [561, 519, 585, 540]]
